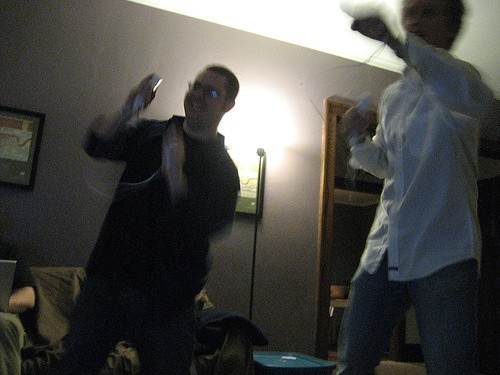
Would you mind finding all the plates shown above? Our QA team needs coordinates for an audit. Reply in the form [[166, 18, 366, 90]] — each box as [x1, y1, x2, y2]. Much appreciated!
[[252, 351, 338, 374]]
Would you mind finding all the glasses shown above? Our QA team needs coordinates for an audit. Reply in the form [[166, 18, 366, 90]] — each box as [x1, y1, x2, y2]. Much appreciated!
[[189, 82, 226, 104]]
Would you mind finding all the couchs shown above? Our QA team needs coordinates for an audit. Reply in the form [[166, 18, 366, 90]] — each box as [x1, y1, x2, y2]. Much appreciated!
[[0, 268, 253, 375]]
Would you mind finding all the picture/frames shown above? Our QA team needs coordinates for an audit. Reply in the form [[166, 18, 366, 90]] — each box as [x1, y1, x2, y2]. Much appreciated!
[[226, 147, 266, 220], [0, 106, 45, 191]]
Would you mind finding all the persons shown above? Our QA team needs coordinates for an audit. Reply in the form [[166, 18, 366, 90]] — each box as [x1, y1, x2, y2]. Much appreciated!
[[333, 0, 493, 375], [0, 238, 35, 375], [56, 64, 241, 375]]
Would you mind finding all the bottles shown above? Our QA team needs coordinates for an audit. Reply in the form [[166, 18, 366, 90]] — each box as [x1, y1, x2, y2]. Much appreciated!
[[132, 73, 163, 114]]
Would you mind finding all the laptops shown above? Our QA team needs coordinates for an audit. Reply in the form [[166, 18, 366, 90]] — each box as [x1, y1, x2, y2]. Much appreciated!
[[0, 260, 17, 312]]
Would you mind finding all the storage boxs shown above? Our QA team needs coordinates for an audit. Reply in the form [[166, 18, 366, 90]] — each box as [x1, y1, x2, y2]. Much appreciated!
[[252, 351, 336, 375], [330, 285, 349, 299]]
